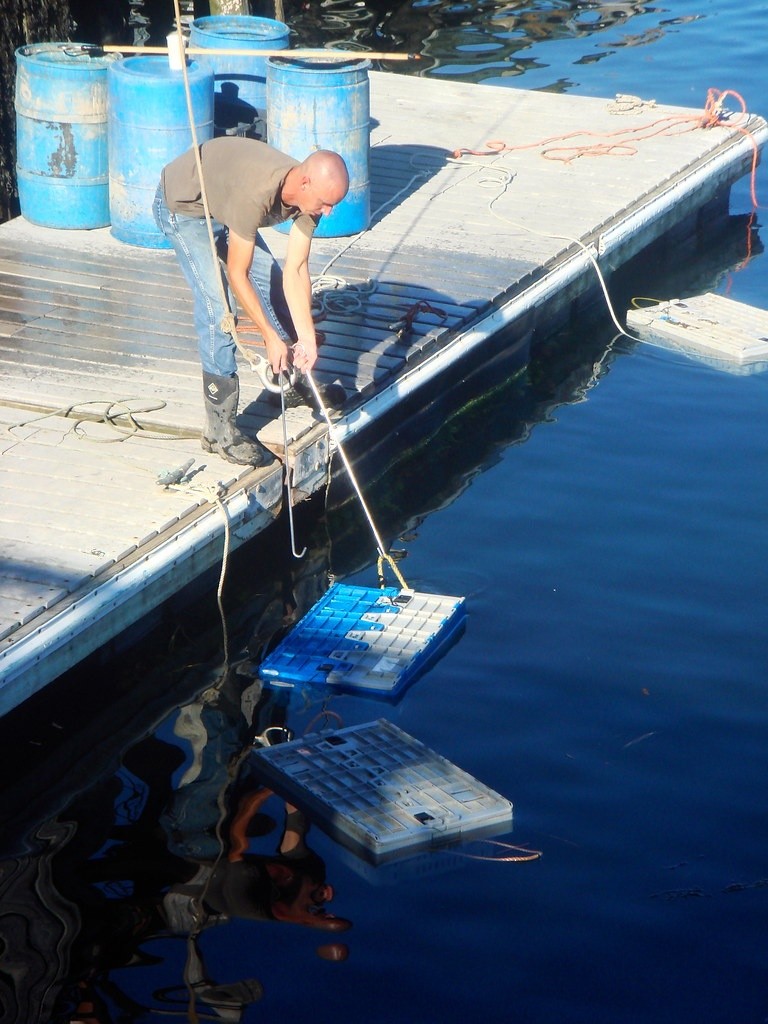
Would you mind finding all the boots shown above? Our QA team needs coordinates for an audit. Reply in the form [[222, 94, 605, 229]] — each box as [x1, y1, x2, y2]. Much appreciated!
[[202, 370, 265, 465], [264, 339, 347, 408]]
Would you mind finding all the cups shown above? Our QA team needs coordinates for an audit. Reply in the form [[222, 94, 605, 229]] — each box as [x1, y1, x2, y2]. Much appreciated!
[[166, 34, 185, 69]]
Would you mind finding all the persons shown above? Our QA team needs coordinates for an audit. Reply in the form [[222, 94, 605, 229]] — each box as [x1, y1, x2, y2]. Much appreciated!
[[154, 137, 349, 466]]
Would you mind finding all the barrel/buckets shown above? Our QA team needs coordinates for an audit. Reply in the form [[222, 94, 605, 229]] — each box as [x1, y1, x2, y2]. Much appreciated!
[[14, 43, 123, 230], [265, 48, 371, 238], [188, 14, 291, 143], [107, 56, 214, 249]]
